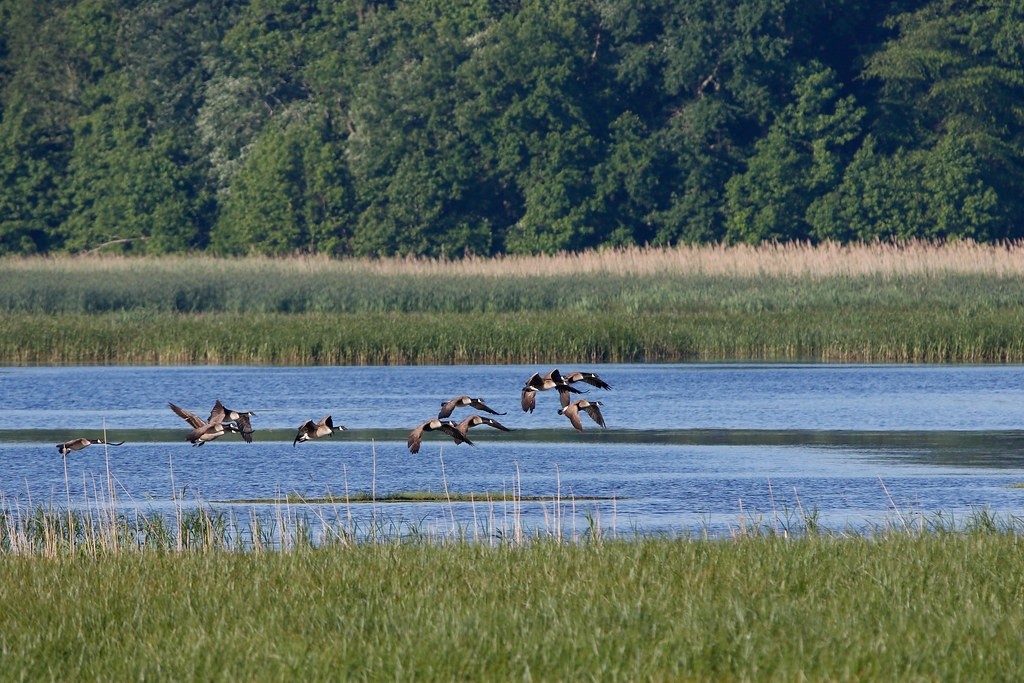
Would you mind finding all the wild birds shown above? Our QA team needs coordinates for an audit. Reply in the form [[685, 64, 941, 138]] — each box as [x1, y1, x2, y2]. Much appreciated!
[[168, 399, 257, 448], [408, 395, 517, 456], [520, 368, 613, 431], [292, 415, 349, 448], [55, 437, 125, 460]]
